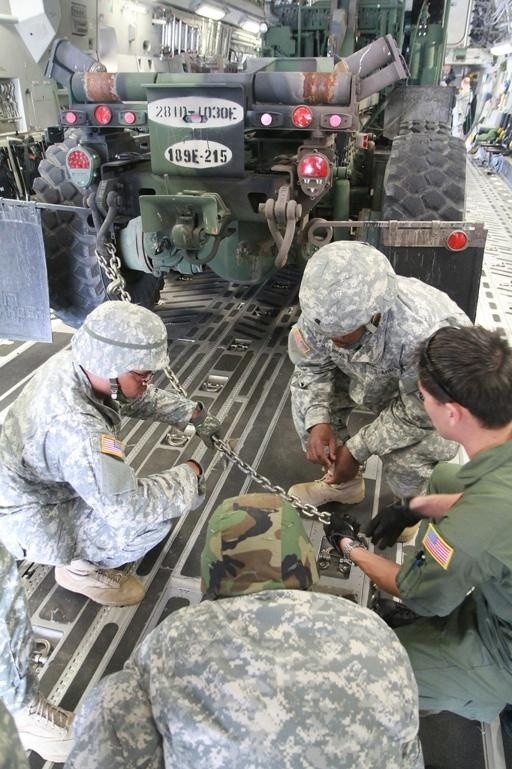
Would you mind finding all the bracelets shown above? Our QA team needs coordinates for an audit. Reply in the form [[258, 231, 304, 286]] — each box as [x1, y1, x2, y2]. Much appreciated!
[[344, 537, 367, 567]]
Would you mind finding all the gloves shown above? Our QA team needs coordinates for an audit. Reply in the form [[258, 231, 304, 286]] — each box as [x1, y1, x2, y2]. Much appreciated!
[[323, 496, 425, 557], [186, 459, 207, 511], [190, 400, 223, 450]]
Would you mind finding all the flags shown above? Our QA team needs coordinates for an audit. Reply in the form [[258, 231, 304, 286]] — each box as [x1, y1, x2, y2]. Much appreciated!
[[293, 328, 310, 353], [422, 524, 453, 569], [102, 434, 122, 457]]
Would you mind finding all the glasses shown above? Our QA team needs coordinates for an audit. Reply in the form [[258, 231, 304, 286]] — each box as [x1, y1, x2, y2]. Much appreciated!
[[129, 371, 156, 386], [422, 325, 469, 409]]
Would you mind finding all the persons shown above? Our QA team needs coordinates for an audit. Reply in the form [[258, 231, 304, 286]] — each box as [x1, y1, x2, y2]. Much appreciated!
[[288, 241, 474, 507], [446, 77, 473, 138], [0, 301, 221, 607], [64, 492, 426, 769], [323, 325, 512, 723], [0, 541, 72, 764]]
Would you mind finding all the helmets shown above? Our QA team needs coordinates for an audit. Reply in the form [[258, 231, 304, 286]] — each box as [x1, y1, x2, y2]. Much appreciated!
[[71, 301, 170, 379], [200, 492, 321, 597], [298, 240, 399, 337]]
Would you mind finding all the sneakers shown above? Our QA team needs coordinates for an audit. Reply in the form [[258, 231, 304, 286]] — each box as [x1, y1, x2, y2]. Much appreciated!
[[287, 462, 366, 508], [54, 560, 146, 606], [373, 598, 421, 628], [501, 705, 512, 738], [12, 691, 77, 763]]
